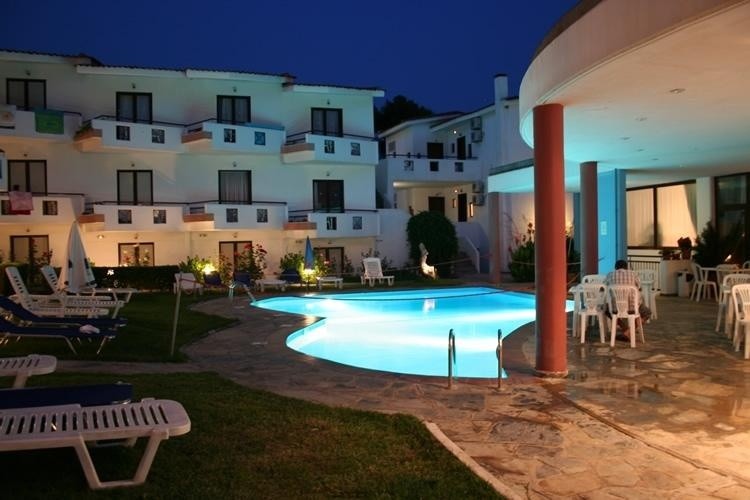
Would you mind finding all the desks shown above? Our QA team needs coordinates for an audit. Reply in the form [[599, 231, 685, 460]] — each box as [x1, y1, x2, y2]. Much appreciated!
[[256, 279, 287, 291], [315, 277, 344, 291]]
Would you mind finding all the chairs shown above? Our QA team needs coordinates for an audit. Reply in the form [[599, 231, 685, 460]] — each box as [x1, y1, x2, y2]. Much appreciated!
[[565, 254, 750, 358], [360, 257, 395, 288], [0, 355, 57, 388], [232, 271, 253, 292], [202, 269, 228, 295], [1, 384, 192, 489], [0, 265, 138, 360]]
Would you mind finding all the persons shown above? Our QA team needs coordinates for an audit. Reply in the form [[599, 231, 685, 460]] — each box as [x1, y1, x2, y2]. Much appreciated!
[[603, 260, 652, 342]]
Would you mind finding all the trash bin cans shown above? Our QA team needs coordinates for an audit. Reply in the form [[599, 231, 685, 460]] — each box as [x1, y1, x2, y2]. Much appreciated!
[[677, 270, 691, 297]]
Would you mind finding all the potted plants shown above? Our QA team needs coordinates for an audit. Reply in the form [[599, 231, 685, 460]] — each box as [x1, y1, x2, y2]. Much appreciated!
[[679, 238, 692, 258]]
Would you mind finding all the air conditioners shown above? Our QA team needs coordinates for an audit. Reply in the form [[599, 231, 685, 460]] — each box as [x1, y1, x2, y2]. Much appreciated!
[[471, 116, 483, 130], [471, 181, 485, 194], [470, 130, 483, 144], [472, 194, 484, 206]]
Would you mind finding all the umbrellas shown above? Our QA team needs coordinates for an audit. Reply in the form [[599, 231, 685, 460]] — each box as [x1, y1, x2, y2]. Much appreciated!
[[304, 235, 316, 291], [57, 220, 97, 297]]
